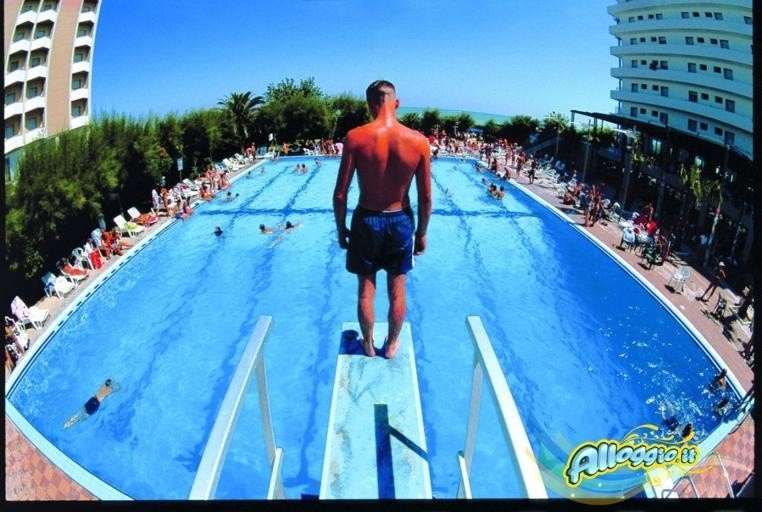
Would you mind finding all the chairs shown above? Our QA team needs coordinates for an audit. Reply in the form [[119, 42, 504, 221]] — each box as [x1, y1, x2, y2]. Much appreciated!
[[4, 295, 50, 366], [480, 140, 614, 219], [151, 152, 252, 213], [40, 206, 158, 298], [666, 267, 690, 294], [620, 212, 675, 270]]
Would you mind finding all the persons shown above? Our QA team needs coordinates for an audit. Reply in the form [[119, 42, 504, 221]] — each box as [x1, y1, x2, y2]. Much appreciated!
[[560, 174, 755, 438], [428, 129, 537, 201], [3, 127, 339, 372], [63, 379, 121, 430], [333, 80, 431, 359]]
